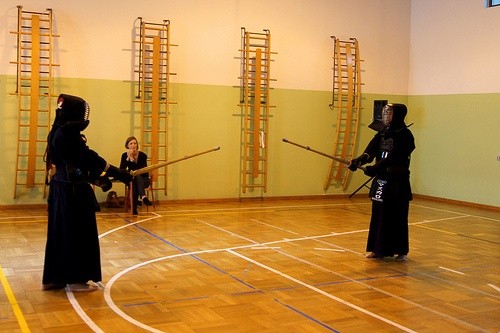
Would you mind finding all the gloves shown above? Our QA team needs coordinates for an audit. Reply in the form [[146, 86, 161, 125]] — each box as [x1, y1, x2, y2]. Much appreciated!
[[347, 153, 368, 172], [364, 166, 378, 177], [89, 175, 113, 192], [104, 164, 137, 186]]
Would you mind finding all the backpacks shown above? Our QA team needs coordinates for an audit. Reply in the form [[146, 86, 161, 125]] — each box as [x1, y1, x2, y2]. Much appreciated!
[[105, 191, 125, 208]]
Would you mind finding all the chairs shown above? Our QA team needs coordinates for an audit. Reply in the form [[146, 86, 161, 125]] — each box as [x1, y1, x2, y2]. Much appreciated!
[[125, 174, 155, 212]]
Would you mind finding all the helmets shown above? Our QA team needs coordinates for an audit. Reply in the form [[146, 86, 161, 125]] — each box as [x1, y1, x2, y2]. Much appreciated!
[[382, 103, 409, 130], [56, 94, 90, 132]]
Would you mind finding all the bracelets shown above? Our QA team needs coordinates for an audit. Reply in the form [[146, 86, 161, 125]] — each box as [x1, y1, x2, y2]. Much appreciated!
[[130, 159, 135, 162]]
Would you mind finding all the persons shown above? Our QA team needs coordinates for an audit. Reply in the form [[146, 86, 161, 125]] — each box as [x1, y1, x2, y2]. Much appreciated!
[[120, 137, 152, 215], [42, 94, 133, 292], [349, 104, 415, 261]]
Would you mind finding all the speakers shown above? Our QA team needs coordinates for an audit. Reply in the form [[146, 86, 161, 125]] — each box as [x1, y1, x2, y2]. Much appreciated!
[[373, 99, 388, 121]]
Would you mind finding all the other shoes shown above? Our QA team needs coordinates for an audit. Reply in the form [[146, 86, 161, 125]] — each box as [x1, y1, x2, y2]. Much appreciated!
[[142, 197, 153, 206], [133, 209, 139, 215]]
